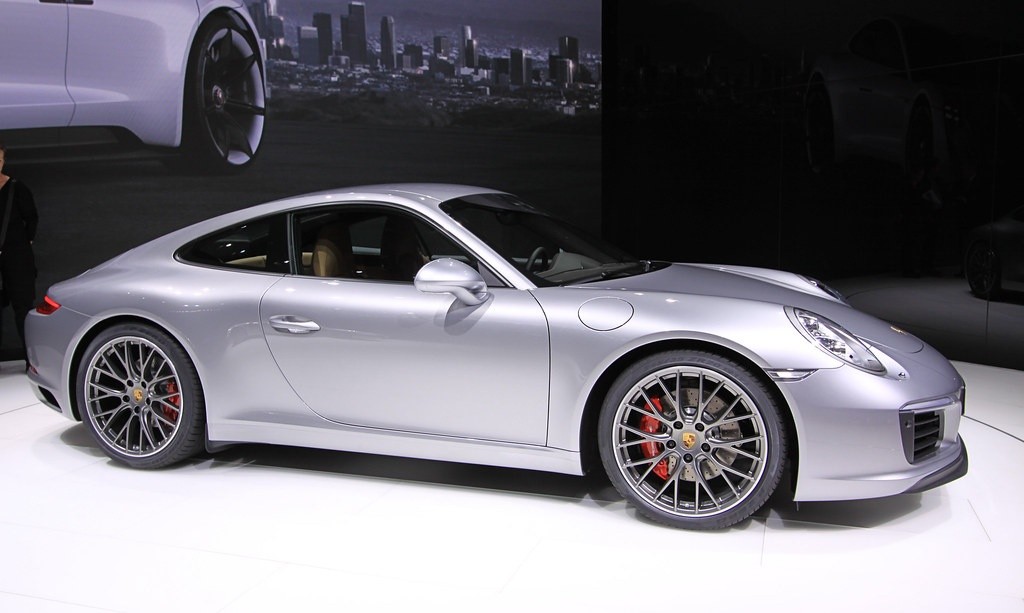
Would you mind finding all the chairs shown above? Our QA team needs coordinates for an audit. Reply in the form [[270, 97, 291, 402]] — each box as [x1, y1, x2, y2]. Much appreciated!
[[379, 218, 431, 282], [312, 239, 360, 281]]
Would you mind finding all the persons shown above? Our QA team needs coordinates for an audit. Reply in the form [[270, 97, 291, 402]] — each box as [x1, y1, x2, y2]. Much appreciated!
[[0, 145, 41, 373]]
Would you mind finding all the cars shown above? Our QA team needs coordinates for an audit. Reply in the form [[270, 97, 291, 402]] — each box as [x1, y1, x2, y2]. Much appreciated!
[[3, 0, 271, 173], [22, 182, 969, 529]]
